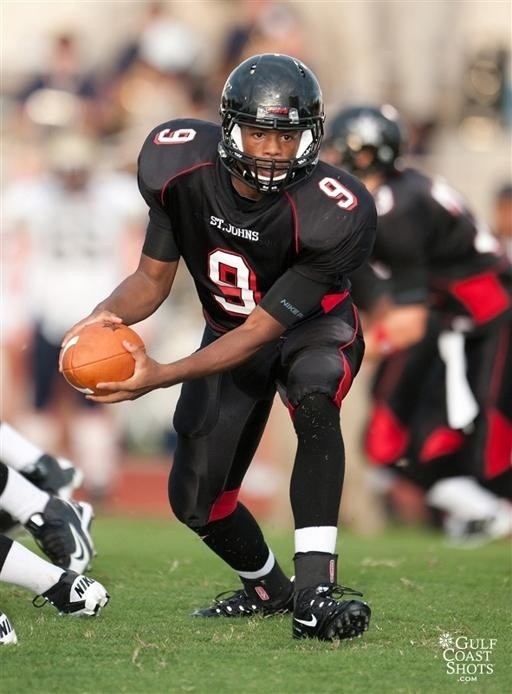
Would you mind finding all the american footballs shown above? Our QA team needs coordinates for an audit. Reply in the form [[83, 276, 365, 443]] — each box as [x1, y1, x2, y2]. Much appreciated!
[[58, 321, 147, 394]]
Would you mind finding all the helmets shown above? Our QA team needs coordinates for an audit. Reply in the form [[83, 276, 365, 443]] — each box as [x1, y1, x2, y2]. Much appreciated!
[[214, 53, 325, 196], [322, 105, 399, 178]]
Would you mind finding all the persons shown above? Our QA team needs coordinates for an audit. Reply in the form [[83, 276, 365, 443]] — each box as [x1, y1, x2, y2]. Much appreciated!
[[0, 1, 512, 644]]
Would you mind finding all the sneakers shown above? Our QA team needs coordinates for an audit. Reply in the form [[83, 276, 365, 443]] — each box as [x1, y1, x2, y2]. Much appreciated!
[[454, 502, 510, 545], [18, 450, 112, 620], [189, 576, 294, 617], [292, 581, 370, 642]]
